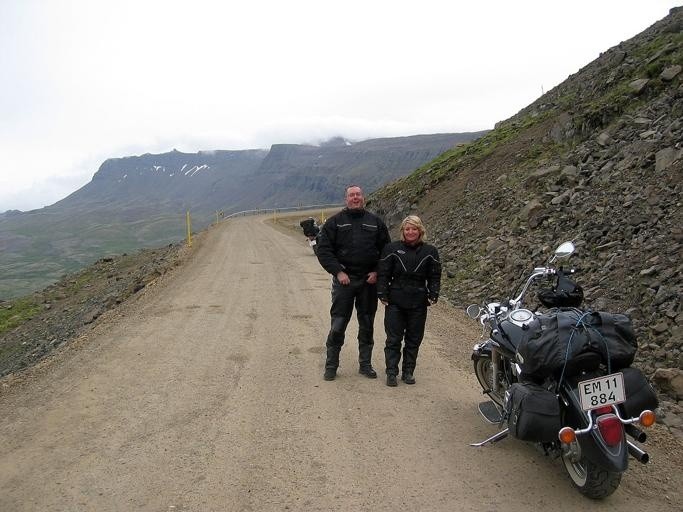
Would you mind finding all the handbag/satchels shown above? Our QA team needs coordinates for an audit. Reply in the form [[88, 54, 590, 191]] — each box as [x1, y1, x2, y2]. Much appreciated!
[[507, 383, 561, 441], [514, 308, 637, 380], [303, 226, 319, 236], [618, 367, 659, 420], [299, 219, 313, 227]]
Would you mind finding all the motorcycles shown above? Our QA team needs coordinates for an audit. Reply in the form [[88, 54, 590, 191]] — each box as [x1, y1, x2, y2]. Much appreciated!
[[465, 241, 660, 501], [306, 216, 326, 252]]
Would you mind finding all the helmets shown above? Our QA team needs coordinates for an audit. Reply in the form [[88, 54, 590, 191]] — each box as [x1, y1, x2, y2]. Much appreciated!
[[537, 276, 584, 309]]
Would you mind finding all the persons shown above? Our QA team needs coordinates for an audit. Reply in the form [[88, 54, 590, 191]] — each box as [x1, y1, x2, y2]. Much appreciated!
[[376, 215, 442, 387], [315, 186, 391, 381]]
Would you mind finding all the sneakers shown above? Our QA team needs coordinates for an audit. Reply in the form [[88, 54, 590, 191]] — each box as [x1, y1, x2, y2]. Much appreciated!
[[360, 366, 377, 378], [402, 372, 415, 384], [387, 375, 397, 386], [323, 369, 336, 380]]
